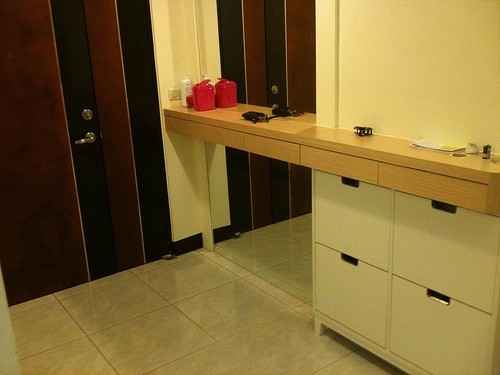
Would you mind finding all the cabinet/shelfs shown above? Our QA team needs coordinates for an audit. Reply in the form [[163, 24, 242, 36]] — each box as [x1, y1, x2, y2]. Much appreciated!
[[312, 170, 500, 375]]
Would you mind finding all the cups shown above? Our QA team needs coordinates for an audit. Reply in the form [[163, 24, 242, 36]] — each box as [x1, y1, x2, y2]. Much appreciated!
[[186, 95, 195, 109]]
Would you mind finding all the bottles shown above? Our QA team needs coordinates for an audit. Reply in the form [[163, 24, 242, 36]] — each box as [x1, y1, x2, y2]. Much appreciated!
[[181, 76, 194, 106]]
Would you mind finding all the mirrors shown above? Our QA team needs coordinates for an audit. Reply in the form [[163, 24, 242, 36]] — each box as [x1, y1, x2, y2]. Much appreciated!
[[197, 2, 316, 307]]
